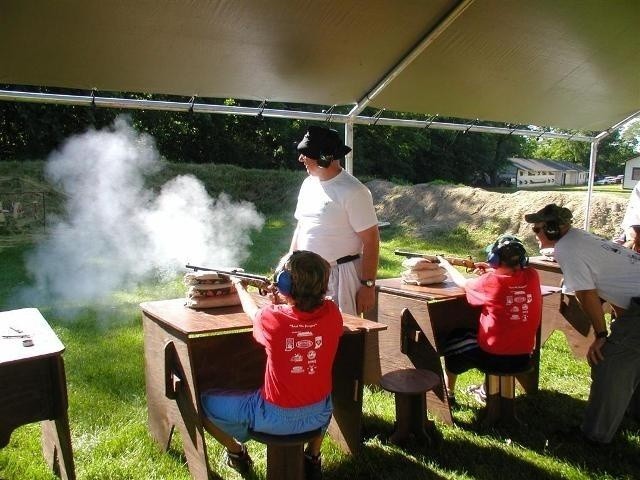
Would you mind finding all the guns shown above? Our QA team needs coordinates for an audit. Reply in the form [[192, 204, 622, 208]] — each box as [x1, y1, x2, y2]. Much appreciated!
[[394, 250, 485, 274], [185, 262, 280, 297]]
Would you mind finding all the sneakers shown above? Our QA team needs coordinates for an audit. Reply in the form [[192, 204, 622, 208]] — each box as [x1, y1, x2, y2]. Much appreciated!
[[466, 383, 487, 408], [445, 386, 456, 402], [225, 442, 253, 471], [303, 447, 322, 476]]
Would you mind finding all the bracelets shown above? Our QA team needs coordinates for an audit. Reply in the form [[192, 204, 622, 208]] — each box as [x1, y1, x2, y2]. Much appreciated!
[[595, 331, 610, 340]]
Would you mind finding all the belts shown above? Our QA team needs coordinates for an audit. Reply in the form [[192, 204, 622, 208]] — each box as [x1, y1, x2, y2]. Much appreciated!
[[337, 251, 361, 267]]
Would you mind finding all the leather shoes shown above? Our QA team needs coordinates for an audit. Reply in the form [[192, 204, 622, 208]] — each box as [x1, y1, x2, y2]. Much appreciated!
[[557, 425, 608, 449]]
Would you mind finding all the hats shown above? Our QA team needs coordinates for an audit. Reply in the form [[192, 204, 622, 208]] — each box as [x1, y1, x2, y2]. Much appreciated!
[[486, 234, 526, 267], [296, 125, 351, 161], [525, 203, 573, 226]]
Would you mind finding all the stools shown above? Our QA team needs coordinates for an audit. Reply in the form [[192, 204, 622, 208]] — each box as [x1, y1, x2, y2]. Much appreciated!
[[243, 427, 322, 480], [475, 364, 536, 437], [377, 366, 444, 449]]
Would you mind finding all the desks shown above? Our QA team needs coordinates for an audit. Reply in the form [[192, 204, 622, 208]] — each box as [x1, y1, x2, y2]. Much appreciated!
[[0, 306, 79, 480], [138, 288, 390, 480], [520, 255, 613, 363], [357, 271, 563, 432]]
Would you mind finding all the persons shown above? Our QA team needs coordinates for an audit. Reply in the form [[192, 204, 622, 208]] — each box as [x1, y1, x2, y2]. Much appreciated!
[[436, 235, 542, 402], [276, 126, 379, 319], [620, 179, 638, 252], [523, 204, 639, 442], [201, 250, 343, 479]]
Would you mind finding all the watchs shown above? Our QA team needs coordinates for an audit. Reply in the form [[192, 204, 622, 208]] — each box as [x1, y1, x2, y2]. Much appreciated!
[[361, 278, 375, 287]]
